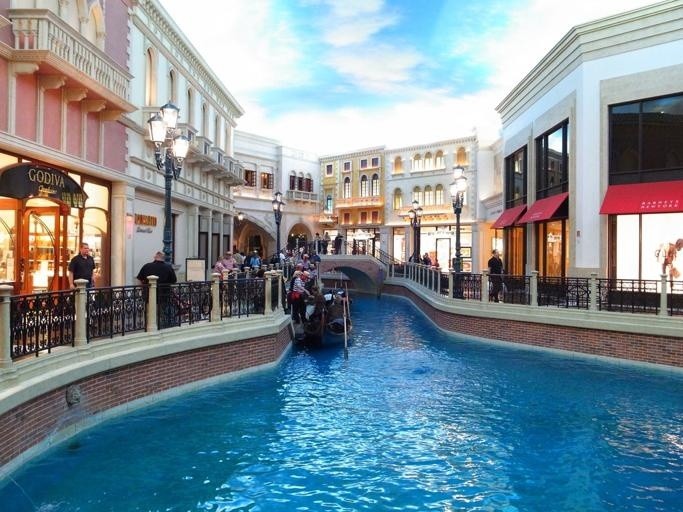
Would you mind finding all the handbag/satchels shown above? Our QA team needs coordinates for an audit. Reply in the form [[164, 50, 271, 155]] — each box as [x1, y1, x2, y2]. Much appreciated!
[[291, 291, 299, 300]]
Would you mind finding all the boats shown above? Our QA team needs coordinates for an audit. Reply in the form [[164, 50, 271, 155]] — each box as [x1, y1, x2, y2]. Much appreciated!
[[303, 289, 353, 350]]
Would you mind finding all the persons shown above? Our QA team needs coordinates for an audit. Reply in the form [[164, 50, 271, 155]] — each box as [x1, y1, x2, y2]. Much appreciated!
[[488, 249, 503, 303], [138, 250, 178, 330], [171, 231, 366, 328], [408, 250, 439, 272], [68, 242, 96, 326], [655, 240, 677, 292]]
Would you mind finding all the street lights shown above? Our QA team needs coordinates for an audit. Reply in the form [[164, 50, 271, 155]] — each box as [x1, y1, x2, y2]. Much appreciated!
[[408, 200, 423, 262], [448, 164, 467, 300], [145, 98, 190, 267], [271, 187, 285, 258]]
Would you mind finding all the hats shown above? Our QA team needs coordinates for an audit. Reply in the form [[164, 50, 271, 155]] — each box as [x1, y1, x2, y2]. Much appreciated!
[[301, 270, 312, 279]]
